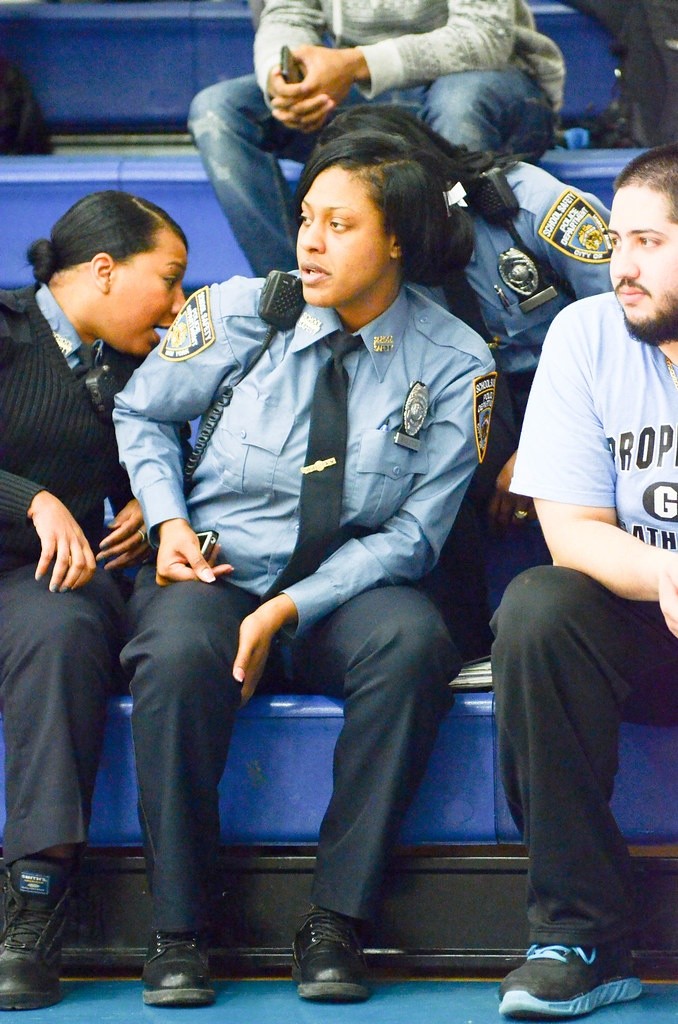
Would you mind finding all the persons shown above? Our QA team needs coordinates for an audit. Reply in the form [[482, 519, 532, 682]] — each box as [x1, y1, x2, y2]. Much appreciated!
[[489, 142, 678, 1019], [188, 0, 564, 277], [1, 189, 190, 1013], [112, 128, 497, 1007], [316, 105, 617, 665]]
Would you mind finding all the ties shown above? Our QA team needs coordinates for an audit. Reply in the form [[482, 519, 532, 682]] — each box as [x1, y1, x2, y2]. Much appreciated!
[[443, 267, 517, 471], [262, 333, 364, 601], [73, 344, 97, 385]]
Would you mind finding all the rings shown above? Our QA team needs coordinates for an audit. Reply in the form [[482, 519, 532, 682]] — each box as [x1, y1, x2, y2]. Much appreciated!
[[137, 528, 148, 543], [513, 510, 528, 520]]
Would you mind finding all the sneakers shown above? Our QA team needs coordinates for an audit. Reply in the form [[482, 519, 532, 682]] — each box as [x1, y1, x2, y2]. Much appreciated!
[[498, 937, 642, 1018]]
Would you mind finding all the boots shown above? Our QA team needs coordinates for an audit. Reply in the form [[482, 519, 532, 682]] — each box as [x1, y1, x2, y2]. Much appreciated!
[[0, 856, 79, 1009]]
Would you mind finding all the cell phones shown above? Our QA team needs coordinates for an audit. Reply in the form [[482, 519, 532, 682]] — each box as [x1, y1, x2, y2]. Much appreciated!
[[186, 530, 219, 568], [281, 45, 303, 85]]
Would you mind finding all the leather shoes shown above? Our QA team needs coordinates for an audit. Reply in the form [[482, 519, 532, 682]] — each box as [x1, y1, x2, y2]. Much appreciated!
[[292, 900, 370, 1001], [143, 928, 213, 1004]]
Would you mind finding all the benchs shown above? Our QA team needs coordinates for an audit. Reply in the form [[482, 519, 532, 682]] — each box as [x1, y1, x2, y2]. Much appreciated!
[[1, 1, 677, 846]]
